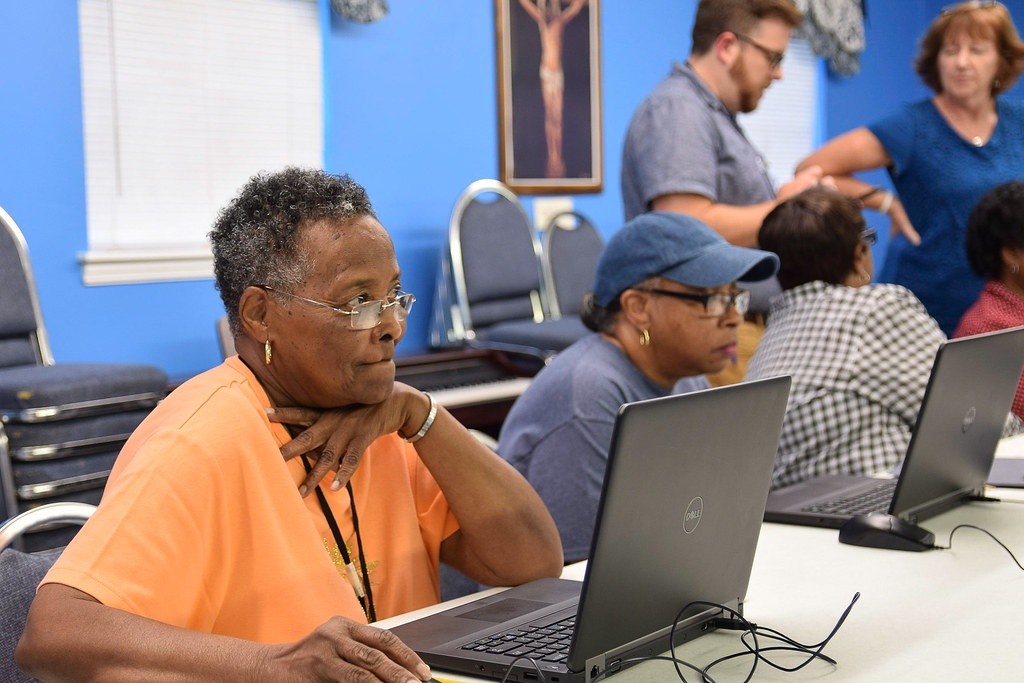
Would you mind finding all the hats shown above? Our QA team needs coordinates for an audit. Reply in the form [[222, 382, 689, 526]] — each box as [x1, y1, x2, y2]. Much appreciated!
[[593, 210, 779, 308]]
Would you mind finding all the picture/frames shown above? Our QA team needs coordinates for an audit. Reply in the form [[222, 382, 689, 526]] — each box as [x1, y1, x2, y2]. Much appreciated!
[[494, 0, 602, 194]]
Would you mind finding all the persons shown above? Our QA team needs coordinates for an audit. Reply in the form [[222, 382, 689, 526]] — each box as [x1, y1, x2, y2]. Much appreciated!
[[15, 166, 564, 683], [950, 181, 1024, 425], [621, 0, 825, 246], [799, 0, 1024, 338], [747, 188, 1024, 491], [478, 210, 781, 590]]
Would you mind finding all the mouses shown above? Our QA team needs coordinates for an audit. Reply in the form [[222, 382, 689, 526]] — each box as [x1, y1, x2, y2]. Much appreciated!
[[839, 511, 935, 552]]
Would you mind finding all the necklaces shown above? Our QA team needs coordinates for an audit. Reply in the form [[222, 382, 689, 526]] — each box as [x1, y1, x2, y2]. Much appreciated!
[[238, 346, 378, 623], [974, 136, 983, 145]]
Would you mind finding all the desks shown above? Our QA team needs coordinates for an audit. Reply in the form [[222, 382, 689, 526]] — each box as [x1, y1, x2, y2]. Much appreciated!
[[348, 403, 1024, 683]]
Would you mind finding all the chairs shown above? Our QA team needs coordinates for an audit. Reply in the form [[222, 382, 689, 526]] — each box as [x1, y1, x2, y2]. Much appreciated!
[[448, 178, 605, 367], [0, 502, 98, 683], [0, 209, 165, 554]]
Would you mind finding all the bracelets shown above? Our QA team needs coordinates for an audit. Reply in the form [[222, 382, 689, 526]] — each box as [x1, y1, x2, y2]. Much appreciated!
[[879, 191, 894, 214], [398, 392, 437, 443]]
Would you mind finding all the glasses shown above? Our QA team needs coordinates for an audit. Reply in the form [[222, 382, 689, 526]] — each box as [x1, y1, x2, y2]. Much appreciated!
[[727, 30, 783, 70], [631, 285, 752, 317], [858, 226, 878, 247], [251, 283, 416, 330]]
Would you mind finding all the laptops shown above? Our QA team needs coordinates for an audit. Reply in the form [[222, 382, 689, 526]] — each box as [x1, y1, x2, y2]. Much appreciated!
[[386, 374, 792, 683], [763, 325, 1024, 529]]
